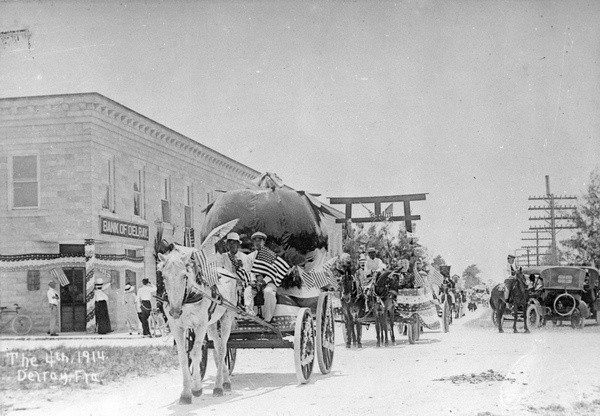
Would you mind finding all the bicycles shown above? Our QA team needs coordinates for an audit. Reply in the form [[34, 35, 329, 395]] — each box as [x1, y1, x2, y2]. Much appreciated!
[[0, 303, 33, 335]]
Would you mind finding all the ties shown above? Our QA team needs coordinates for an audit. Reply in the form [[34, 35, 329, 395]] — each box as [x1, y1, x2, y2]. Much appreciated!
[[511, 265, 515, 271]]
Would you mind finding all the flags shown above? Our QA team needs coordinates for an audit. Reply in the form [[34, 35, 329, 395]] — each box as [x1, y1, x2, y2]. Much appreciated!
[[414, 270, 424, 287], [227, 253, 251, 289], [250, 246, 290, 288], [431, 267, 444, 288], [295, 256, 340, 292], [195, 246, 219, 288]]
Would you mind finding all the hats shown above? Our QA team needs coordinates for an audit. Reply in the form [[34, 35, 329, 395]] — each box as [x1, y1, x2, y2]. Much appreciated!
[[251, 232, 267, 240], [507, 255, 515, 259], [123, 285, 135, 293], [368, 248, 376, 253], [223, 232, 242, 244], [48, 280, 56, 285]]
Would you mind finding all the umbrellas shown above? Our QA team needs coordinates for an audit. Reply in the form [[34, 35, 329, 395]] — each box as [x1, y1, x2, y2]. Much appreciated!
[[199, 218, 240, 245]]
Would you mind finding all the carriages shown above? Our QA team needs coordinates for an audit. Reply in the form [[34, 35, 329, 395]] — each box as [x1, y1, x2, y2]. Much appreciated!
[[334, 267, 451, 348], [492, 270, 540, 331], [152, 250, 335, 405]]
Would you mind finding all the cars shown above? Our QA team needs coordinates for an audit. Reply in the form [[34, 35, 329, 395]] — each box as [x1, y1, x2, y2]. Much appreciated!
[[535, 266, 600, 329]]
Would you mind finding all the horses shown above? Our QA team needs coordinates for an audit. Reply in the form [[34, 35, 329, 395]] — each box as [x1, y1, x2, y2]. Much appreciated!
[[440, 282, 452, 324], [367, 268, 396, 347], [335, 272, 365, 348], [155, 251, 238, 405], [489, 266, 530, 333]]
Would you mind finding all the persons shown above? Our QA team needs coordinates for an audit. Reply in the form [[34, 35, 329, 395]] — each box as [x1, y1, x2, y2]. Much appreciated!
[[137, 279, 156, 336], [221, 232, 247, 311], [504, 254, 519, 302], [461, 290, 467, 317], [47, 280, 61, 335], [364, 248, 386, 278], [355, 259, 374, 290], [528, 275, 542, 290], [123, 284, 142, 336], [94, 284, 108, 333], [245, 231, 277, 323]]
